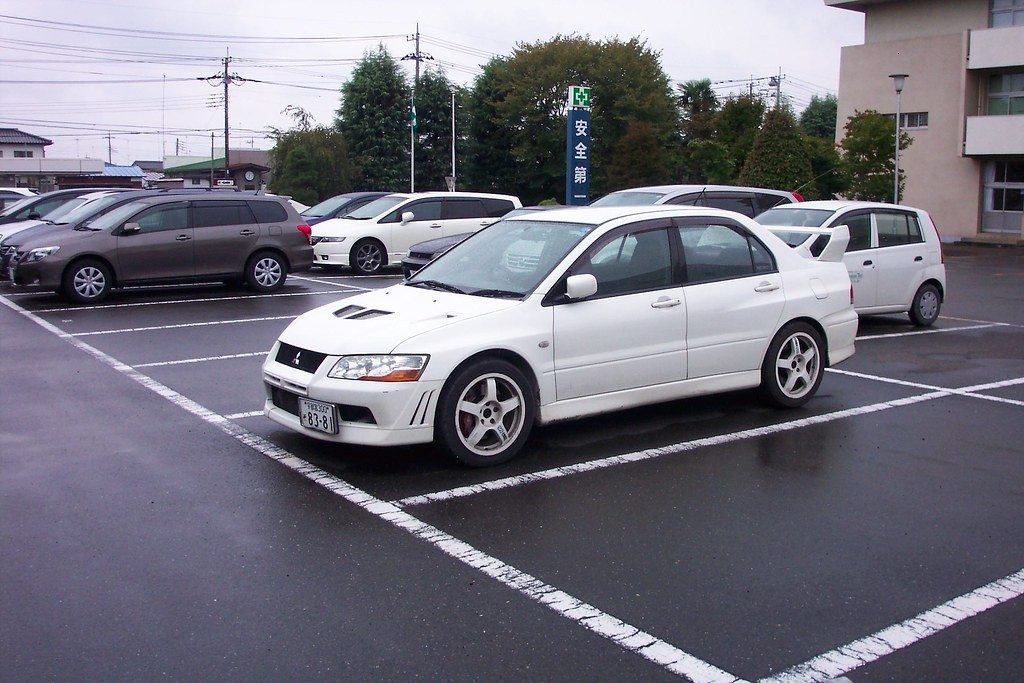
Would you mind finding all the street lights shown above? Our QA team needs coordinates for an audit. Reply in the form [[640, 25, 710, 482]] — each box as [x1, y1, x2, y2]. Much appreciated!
[[888, 73, 910, 203]]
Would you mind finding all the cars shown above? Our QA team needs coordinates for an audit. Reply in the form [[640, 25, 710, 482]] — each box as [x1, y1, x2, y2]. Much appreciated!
[[400, 206, 570, 282], [751, 199, 948, 328], [261, 204, 861, 466]]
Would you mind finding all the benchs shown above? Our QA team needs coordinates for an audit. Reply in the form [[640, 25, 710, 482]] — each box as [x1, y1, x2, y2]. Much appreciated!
[[684, 245, 768, 282], [879, 233, 919, 246]]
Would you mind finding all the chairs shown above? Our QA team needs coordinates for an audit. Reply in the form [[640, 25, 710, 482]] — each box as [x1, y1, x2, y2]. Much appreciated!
[[609, 239, 666, 293], [526, 234, 593, 294]]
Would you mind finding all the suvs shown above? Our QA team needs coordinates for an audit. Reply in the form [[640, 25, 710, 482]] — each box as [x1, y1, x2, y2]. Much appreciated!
[[298, 192, 397, 228], [501, 182, 814, 288], [310, 191, 524, 276], [0, 182, 314, 307]]
